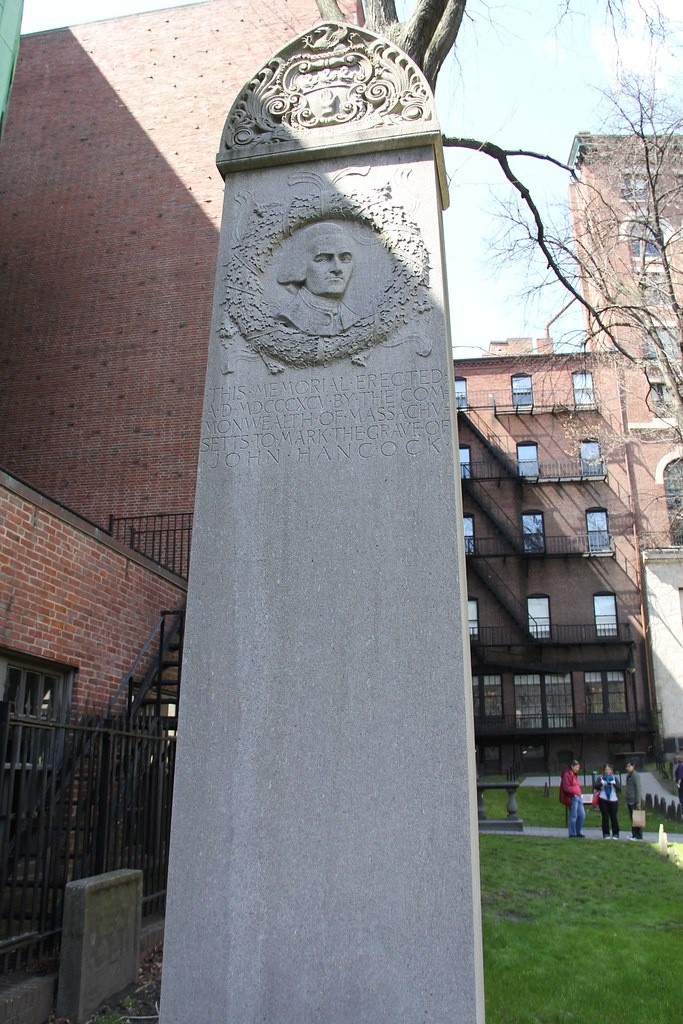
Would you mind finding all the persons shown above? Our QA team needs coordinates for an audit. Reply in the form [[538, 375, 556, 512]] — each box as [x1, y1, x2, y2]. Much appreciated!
[[559, 760, 585, 837], [675, 756, 683, 814], [595, 764, 621, 839], [626, 761, 643, 841], [276, 222, 362, 336]]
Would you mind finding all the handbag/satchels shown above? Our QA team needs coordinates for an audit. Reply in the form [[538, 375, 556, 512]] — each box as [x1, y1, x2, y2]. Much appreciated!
[[593, 776, 602, 807], [632, 807, 645, 828]]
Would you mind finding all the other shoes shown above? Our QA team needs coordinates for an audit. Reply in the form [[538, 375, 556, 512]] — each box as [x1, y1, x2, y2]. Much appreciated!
[[627, 836, 642, 842], [577, 833, 584, 837], [605, 836, 611, 840], [613, 837, 618, 840], [569, 835, 578, 837]]
